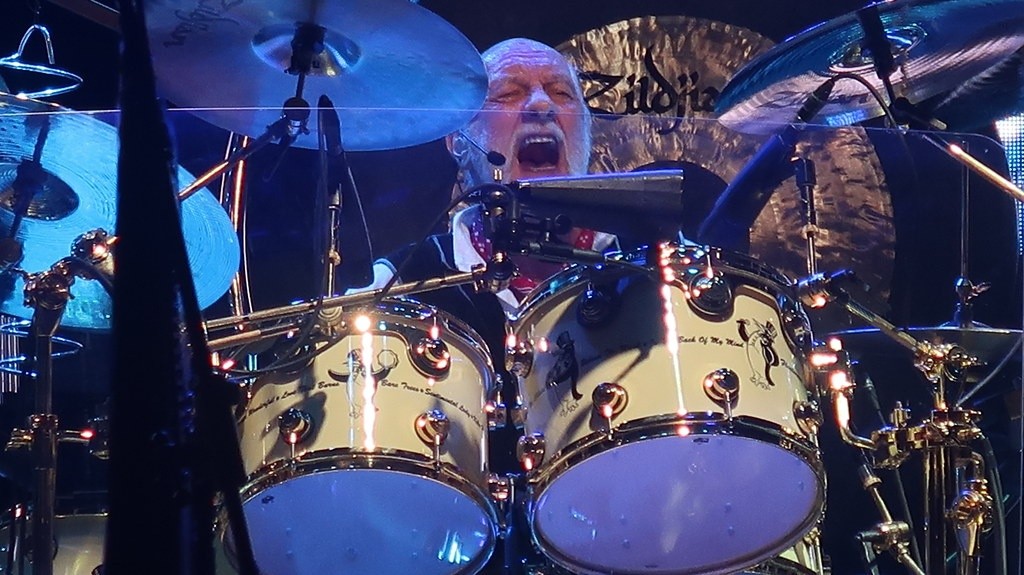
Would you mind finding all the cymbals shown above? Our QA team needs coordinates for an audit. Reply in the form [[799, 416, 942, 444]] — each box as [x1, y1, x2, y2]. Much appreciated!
[[136, 0, 487, 154], [715, 0, 1022, 134], [0, 94, 245, 332], [817, 316, 1024, 349]]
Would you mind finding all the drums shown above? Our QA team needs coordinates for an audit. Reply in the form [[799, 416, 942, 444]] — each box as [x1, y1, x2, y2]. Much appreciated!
[[503, 239, 832, 573], [231, 291, 505, 573]]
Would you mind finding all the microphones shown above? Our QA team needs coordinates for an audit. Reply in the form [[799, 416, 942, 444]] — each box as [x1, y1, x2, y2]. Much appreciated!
[[458, 129, 506, 166], [695, 122, 800, 254]]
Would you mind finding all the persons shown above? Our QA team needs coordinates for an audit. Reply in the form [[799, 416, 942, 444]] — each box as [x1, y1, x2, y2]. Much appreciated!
[[290, 38, 697, 575]]
